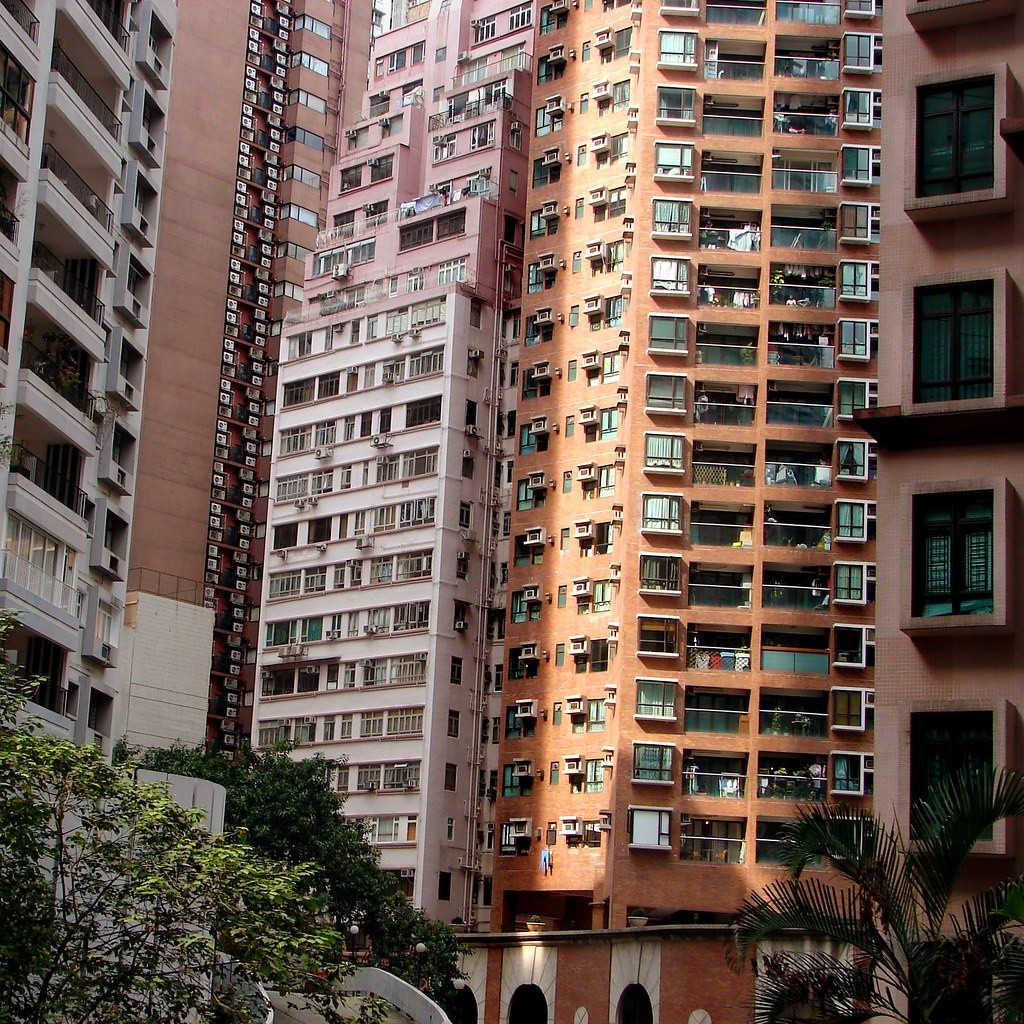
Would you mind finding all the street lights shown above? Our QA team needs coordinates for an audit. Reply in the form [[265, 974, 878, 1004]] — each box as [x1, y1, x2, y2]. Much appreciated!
[[454, 979, 465, 1024], [350, 925, 359, 963], [415, 943, 425, 990]]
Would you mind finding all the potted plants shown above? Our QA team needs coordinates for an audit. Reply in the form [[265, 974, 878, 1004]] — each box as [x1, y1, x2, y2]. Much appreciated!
[[448, 916, 468, 933], [526, 915, 545, 932], [628, 908, 649, 927]]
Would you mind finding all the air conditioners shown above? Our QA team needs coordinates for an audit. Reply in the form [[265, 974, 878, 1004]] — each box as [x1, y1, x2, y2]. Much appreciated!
[[200, 1, 632, 878]]
